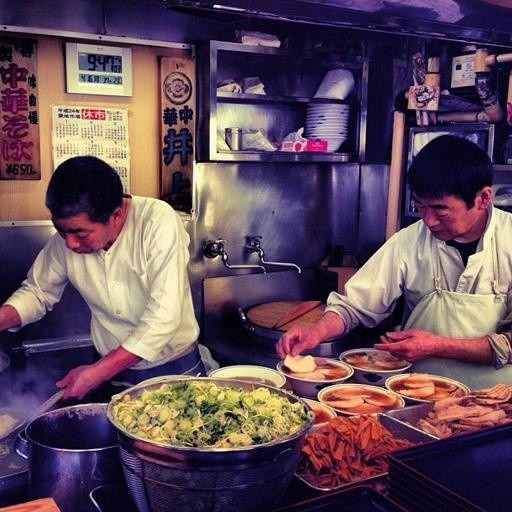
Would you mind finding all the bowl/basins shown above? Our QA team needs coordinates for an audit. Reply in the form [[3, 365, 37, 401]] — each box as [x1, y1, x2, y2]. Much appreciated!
[[303, 103, 351, 154], [208, 344, 472, 430]]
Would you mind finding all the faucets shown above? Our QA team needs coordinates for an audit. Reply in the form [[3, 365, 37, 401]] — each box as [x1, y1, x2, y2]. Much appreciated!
[[205, 238, 266, 275], [243, 234, 303, 275]]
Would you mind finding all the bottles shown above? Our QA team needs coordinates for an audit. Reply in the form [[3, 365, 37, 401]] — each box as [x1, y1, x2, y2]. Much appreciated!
[[225, 127, 243, 151]]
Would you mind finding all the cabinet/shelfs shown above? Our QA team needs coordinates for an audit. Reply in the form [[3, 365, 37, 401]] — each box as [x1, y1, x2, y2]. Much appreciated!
[[203, 38, 368, 163]]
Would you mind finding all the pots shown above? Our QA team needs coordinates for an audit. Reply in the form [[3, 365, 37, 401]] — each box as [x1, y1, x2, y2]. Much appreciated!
[[12, 399, 121, 511], [108, 374, 315, 512], [237, 302, 347, 343]]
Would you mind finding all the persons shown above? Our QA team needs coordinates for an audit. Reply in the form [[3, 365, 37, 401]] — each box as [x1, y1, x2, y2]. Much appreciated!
[[0, 154, 207, 403], [274, 133, 511, 371]]
